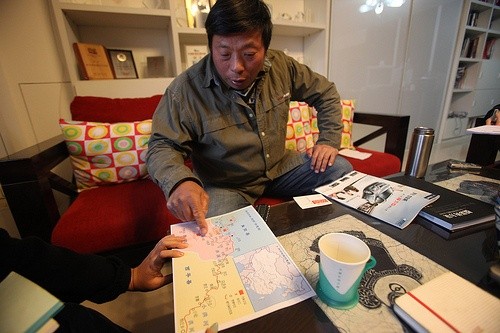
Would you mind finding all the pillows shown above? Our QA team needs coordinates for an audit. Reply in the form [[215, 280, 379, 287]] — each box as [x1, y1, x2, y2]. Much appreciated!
[[58, 117, 155, 192], [282, 99, 359, 156]]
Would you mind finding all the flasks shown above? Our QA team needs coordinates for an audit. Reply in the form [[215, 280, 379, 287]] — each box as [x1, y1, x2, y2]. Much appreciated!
[[405, 126, 435, 178]]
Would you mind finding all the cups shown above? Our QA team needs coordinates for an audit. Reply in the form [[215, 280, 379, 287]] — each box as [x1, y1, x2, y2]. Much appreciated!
[[316, 232, 376, 310], [282, 11, 305, 22]]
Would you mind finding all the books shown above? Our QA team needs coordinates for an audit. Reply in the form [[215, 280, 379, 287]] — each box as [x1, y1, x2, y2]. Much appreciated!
[[393, 270, 500, 333], [415, 215, 496, 241], [312, 169, 440, 229], [385, 174, 497, 234]]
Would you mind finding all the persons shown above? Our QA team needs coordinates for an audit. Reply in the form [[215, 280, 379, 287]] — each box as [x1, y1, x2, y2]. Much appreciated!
[[0, 228, 189, 333], [145, 0, 352, 237]]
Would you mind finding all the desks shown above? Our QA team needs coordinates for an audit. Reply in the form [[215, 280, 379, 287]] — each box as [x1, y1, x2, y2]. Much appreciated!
[[166, 159, 499, 333]]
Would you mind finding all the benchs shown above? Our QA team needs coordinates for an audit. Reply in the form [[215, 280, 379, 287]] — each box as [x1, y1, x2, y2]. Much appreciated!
[[0, 95, 411, 270]]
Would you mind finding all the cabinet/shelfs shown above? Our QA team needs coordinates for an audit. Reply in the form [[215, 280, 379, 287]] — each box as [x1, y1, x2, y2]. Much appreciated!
[[393, 0, 500, 171], [51, 0, 331, 103]]
